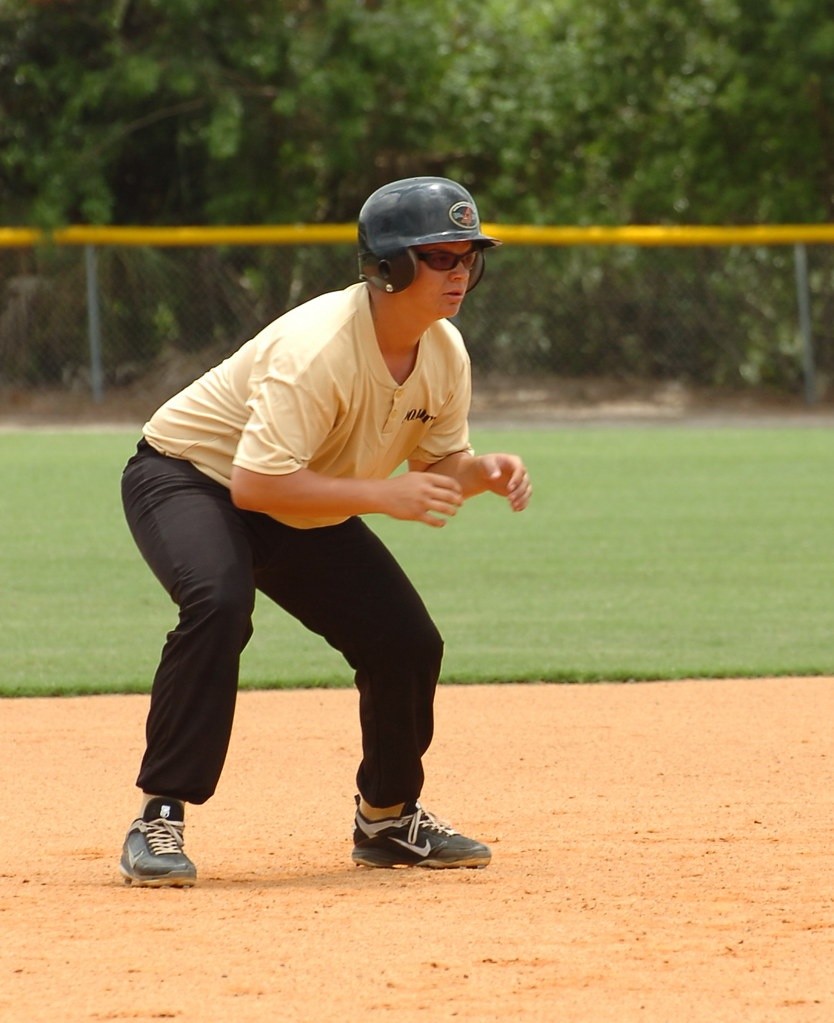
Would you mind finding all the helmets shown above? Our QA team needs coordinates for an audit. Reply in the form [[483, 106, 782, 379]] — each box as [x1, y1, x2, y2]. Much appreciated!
[[358, 176, 502, 293]]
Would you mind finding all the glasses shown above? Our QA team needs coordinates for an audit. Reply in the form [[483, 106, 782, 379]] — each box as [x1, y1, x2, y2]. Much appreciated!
[[418, 248, 480, 271]]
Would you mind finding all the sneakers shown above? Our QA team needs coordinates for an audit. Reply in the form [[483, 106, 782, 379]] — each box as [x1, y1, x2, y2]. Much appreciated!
[[352, 793, 492, 869], [119, 797, 196, 888]]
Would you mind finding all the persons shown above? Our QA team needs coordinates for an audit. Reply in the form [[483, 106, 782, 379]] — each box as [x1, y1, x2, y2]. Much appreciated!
[[121, 175, 531, 886]]
